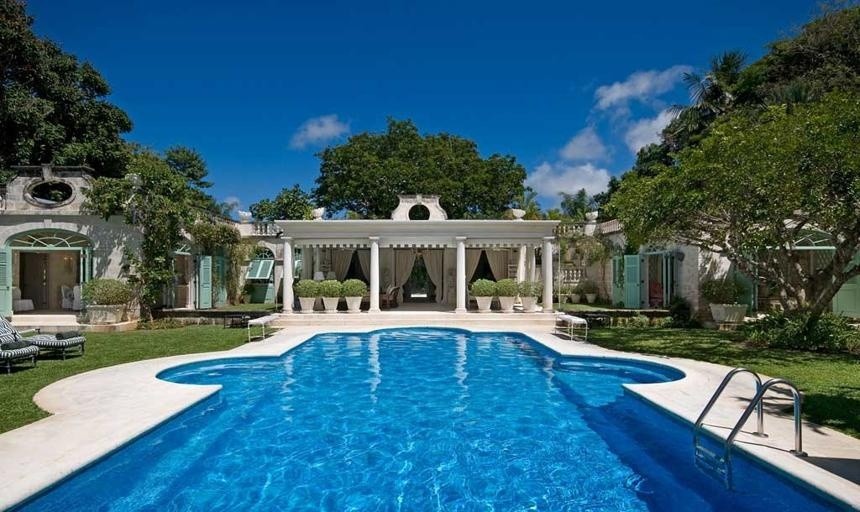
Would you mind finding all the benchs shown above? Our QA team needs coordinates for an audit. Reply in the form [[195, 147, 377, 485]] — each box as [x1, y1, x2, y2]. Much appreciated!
[[247, 315, 281, 343], [555, 315, 588, 342]]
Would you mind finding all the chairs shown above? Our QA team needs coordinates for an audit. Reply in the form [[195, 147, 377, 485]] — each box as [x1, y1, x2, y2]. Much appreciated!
[[61, 283, 82, 313], [0, 312, 88, 374], [12, 287, 34, 314]]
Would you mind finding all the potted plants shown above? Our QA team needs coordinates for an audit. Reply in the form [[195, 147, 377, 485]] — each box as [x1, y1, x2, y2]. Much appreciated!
[[80, 279, 134, 325], [292, 278, 368, 315], [241, 284, 255, 304], [556, 284, 600, 304], [471, 278, 544, 313]]
